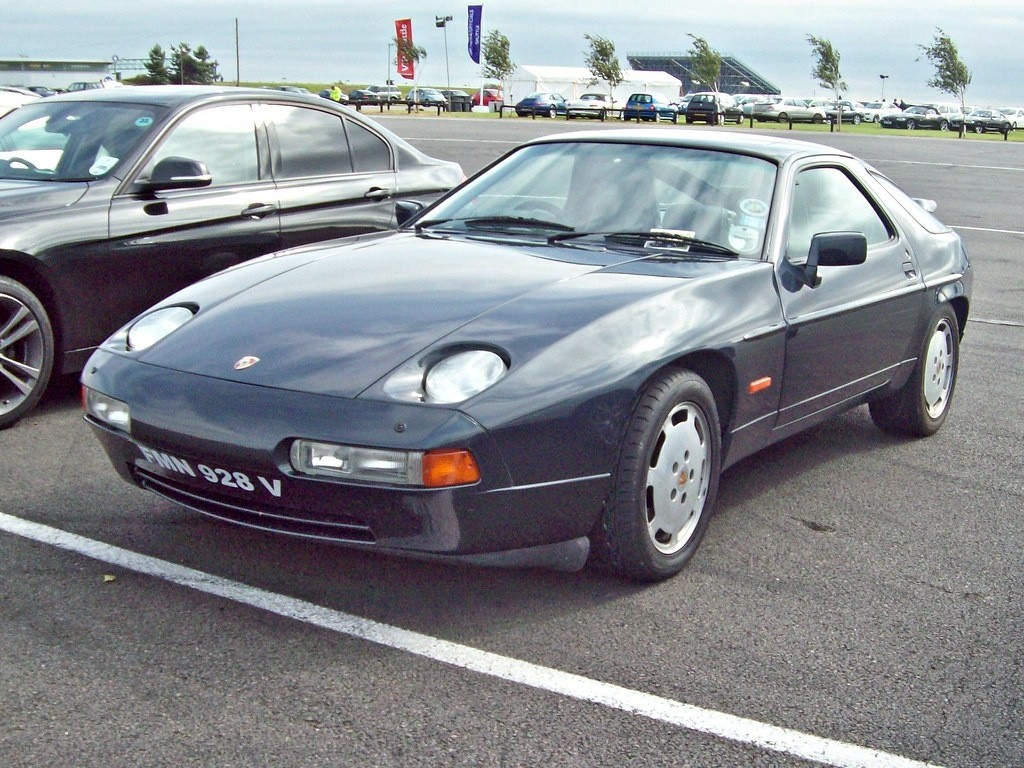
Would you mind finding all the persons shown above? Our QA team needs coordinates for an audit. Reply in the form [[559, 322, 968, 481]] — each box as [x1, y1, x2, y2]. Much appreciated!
[[330, 85, 341, 101], [874, 100, 879, 109], [892, 99, 898, 108], [839, 95, 842, 100], [882, 99, 885, 102], [899, 99, 906, 111]]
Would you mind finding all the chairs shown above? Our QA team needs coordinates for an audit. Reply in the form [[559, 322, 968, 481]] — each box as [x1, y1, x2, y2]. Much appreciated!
[[564, 158, 660, 234], [662, 203, 730, 246]]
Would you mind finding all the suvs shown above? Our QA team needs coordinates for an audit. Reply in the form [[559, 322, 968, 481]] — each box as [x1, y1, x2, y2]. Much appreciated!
[[365, 85, 402, 105]]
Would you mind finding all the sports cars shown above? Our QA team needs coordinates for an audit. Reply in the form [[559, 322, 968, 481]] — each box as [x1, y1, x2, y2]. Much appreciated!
[[81, 128, 971, 587], [566, 94, 626, 121], [948, 109, 1012, 134], [514, 92, 570, 119], [880, 106, 950, 132]]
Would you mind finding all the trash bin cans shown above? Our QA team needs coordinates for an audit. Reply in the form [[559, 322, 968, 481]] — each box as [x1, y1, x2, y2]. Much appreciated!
[[451, 95, 472, 112]]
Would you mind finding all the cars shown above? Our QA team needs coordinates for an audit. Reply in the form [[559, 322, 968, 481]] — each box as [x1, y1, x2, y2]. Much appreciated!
[[405, 88, 447, 108], [622, 93, 680, 125], [278, 86, 318, 98], [319, 89, 348, 104], [0, 87, 472, 430], [472, 88, 503, 106], [687, 94, 744, 126], [678, 92, 1024, 129], [752, 97, 826, 125], [29, 79, 124, 98], [346, 90, 380, 106], [441, 90, 473, 106], [0, 89, 121, 174]]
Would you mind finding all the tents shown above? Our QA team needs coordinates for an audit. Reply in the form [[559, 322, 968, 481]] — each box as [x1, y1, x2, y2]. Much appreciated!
[[503, 64, 682, 115]]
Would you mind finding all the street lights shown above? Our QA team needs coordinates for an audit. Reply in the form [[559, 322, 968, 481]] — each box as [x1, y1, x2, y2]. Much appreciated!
[[879, 74, 889, 101], [435, 15, 452, 111], [387, 42, 394, 110]]
